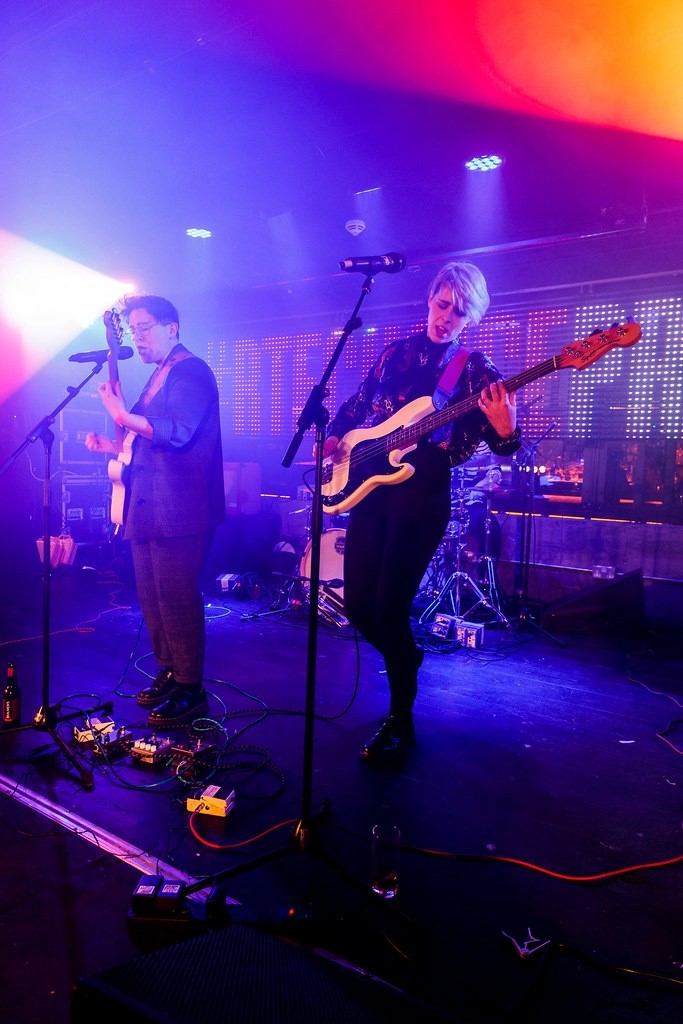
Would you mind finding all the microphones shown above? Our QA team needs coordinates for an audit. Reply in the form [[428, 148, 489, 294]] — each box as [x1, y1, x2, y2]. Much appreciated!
[[328, 580, 340, 587], [68, 346, 134, 363], [338, 253, 407, 274]]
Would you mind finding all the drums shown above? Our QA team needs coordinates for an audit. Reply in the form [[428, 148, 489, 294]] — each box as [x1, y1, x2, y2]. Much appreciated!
[[295, 527, 352, 629]]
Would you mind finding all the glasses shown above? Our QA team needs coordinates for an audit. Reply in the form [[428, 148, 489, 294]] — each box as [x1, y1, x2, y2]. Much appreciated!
[[124, 322, 162, 335]]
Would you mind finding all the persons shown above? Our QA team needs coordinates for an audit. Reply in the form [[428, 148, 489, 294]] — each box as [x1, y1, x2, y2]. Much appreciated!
[[86, 296, 228, 728], [465, 467, 502, 586], [313, 260, 521, 761]]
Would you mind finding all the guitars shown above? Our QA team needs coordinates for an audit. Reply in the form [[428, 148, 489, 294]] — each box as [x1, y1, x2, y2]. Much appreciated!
[[101, 303, 143, 530], [313, 318, 644, 520]]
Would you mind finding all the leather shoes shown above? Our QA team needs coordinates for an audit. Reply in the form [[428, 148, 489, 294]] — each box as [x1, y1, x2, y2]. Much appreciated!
[[136, 668, 175, 706], [148, 681, 207, 726]]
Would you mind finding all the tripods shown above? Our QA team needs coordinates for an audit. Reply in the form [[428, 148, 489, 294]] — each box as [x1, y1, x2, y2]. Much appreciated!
[[0, 363, 114, 786], [240, 572, 347, 632], [181, 275, 394, 912], [418, 423, 568, 649]]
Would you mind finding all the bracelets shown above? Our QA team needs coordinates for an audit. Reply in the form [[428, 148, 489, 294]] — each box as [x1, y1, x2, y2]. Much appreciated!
[[115, 410, 129, 426]]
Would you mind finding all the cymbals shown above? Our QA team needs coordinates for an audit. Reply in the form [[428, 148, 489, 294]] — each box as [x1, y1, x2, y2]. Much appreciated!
[[466, 484, 522, 494]]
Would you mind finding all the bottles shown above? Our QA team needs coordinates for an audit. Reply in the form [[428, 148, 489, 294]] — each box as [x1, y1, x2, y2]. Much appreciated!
[[3, 662, 21, 725]]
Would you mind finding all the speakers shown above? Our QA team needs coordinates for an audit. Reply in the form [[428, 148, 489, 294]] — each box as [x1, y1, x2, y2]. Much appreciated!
[[71, 924, 467, 1024], [543, 568, 644, 636]]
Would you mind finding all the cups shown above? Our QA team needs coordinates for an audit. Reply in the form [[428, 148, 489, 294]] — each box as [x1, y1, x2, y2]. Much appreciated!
[[370, 824, 405, 895]]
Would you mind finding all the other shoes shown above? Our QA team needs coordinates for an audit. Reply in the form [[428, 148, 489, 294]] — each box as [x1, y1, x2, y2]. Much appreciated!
[[362, 708, 416, 764]]
